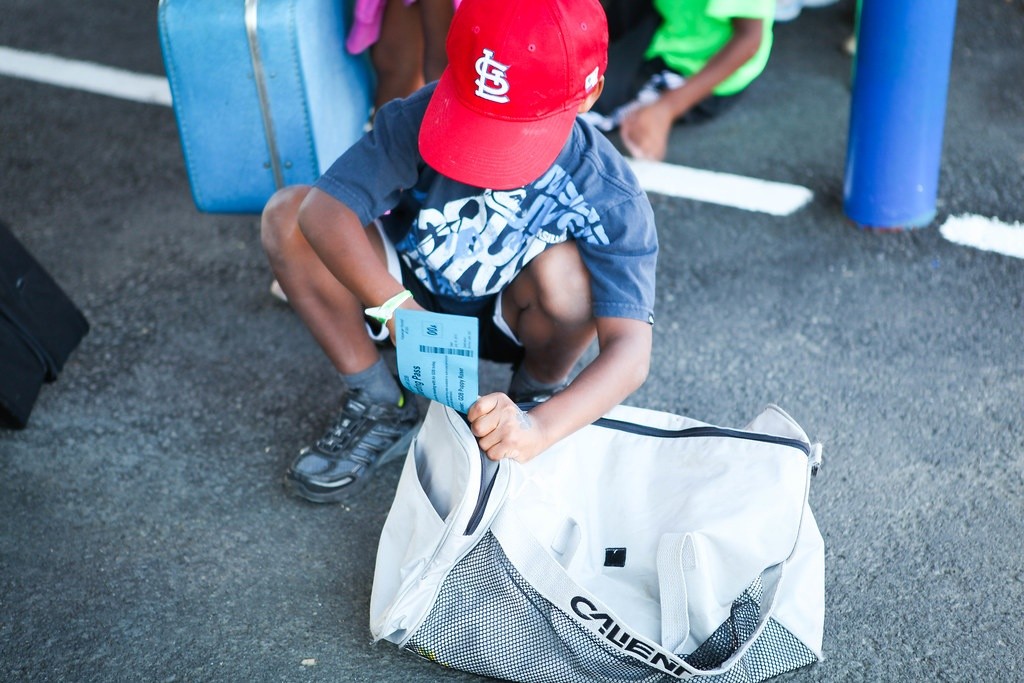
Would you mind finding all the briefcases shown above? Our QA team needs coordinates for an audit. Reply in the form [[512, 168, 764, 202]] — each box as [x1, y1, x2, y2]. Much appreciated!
[[154, 0, 376, 214]]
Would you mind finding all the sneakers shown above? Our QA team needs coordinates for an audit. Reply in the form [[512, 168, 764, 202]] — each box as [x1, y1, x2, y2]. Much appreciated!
[[504, 361, 571, 415], [282, 373, 424, 504]]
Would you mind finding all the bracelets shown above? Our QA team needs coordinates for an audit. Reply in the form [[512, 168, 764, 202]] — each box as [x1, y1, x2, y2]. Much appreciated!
[[363, 289, 414, 342]]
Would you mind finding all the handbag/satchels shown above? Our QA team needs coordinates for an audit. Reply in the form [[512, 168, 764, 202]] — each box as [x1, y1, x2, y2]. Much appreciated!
[[368, 391, 827, 683], [0, 223, 90, 430]]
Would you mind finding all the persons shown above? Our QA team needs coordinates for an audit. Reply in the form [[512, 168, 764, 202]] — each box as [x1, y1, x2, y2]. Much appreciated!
[[342, 0, 452, 122], [579, 1, 780, 165], [259, 0, 661, 502]]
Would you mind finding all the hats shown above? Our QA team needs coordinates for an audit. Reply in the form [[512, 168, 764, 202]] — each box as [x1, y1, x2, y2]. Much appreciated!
[[416, 0, 610, 191]]
